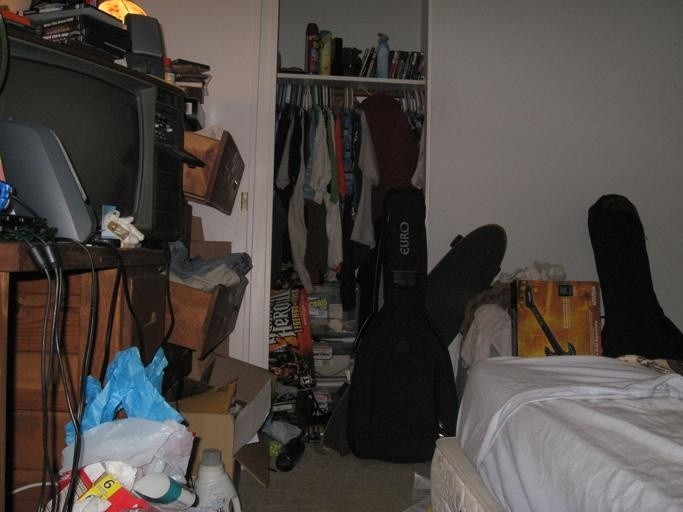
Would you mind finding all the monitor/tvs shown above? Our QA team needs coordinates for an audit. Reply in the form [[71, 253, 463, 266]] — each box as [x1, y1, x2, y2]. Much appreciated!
[[0, 21, 187, 244]]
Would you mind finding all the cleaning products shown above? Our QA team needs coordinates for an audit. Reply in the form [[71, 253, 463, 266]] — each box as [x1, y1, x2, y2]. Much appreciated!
[[377, 33, 389, 77]]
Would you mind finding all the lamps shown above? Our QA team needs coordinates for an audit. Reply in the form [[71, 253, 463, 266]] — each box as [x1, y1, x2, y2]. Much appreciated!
[[95, 0, 148, 25]]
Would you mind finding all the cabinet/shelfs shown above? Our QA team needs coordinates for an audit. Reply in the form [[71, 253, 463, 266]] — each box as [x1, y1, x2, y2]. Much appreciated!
[[0, 130, 247, 512], [264, 0, 432, 387]]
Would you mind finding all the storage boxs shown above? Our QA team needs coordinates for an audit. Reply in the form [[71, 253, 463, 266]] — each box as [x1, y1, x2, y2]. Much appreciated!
[[307, 284, 361, 339], [180, 355, 273, 480]]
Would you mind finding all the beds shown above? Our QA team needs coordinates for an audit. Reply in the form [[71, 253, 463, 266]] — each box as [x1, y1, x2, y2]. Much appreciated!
[[429, 354, 683, 512]]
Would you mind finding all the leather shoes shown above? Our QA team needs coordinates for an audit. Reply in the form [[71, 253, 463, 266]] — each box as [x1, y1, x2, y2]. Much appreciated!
[[276, 438, 305, 472]]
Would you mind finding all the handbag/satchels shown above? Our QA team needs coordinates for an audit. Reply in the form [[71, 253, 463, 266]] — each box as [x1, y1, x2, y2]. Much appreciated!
[[587, 194, 683, 362]]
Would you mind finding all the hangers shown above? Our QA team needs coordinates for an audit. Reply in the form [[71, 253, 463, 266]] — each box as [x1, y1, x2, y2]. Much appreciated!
[[276, 79, 425, 113]]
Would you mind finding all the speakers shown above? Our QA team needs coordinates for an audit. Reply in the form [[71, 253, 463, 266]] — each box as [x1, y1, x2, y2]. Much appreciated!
[[0, 120, 97, 242], [124, 13, 166, 79]]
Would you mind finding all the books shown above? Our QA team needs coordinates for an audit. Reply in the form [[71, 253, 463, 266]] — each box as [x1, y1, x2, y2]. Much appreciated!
[[0, 1, 211, 105], [302, 22, 425, 81]]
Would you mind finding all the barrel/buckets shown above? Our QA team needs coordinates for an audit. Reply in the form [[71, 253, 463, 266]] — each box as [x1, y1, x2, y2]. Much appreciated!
[[195, 449, 242, 512]]
